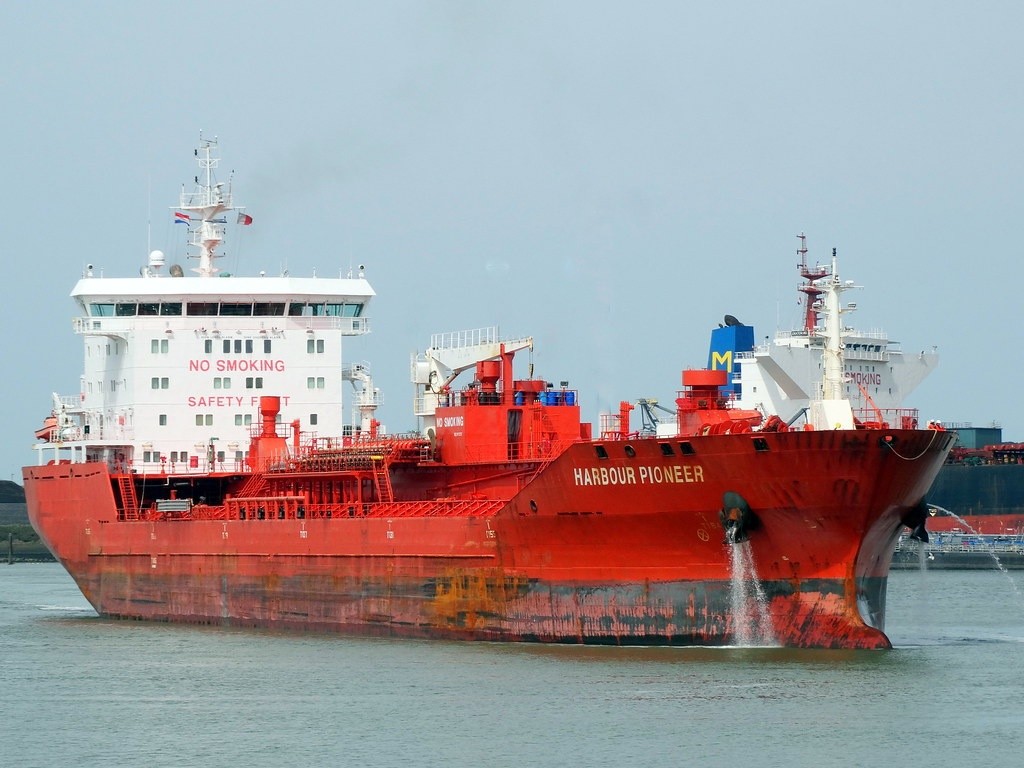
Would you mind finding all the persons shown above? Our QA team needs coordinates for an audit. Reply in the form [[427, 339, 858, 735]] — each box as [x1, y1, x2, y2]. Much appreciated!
[[936, 420, 945, 430], [928, 419, 936, 429]]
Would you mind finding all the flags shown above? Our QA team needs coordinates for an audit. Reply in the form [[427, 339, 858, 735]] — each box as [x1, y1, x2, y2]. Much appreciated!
[[174, 212, 191, 226], [237, 213, 252, 224]]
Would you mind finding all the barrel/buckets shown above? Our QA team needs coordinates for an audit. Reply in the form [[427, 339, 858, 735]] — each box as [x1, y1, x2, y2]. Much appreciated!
[[478, 392, 488, 406], [539, 391, 548, 406], [547, 391, 557, 406], [514, 391, 524, 406], [556, 392, 563, 406], [565, 392, 574, 406], [489, 392, 498, 406]]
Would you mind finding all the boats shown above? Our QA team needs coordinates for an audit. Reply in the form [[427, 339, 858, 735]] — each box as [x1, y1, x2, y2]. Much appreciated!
[[902, 421, 1024, 550], [20, 127, 959, 651]]
